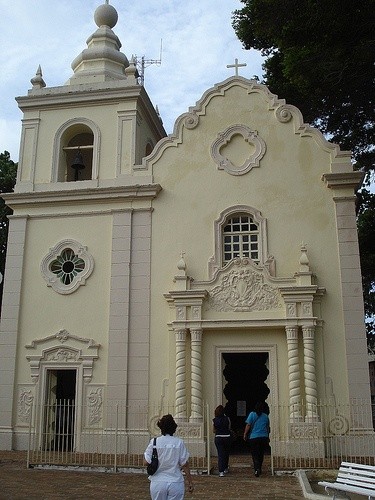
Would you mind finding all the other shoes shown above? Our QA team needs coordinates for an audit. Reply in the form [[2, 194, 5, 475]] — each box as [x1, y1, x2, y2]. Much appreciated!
[[224, 470, 229, 473], [255, 470, 258, 477], [219, 472, 224, 477]]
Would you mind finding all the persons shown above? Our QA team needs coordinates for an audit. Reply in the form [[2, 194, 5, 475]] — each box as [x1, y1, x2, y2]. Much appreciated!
[[243, 402, 269, 477], [212, 405, 231, 476], [144, 414, 194, 500], [230, 426, 249, 451]]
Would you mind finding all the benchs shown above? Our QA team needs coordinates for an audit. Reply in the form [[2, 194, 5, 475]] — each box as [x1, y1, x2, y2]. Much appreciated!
[[318, 461, 375, 500]]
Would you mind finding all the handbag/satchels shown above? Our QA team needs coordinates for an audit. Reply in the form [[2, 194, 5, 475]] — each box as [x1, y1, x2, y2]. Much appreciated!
[[147, 437, 159, 475]]
